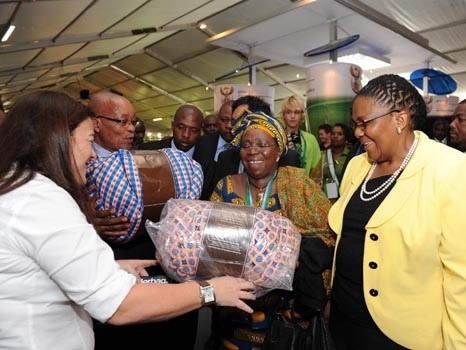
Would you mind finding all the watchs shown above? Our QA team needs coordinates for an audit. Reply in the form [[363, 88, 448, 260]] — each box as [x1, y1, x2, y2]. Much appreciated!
[[189, 277, 218, 308]]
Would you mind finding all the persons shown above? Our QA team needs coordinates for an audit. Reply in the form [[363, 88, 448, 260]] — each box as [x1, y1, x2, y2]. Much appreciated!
[[0, 51, 466, 350], [0, 90, 258, 350], [326, 73, 465, 350], [76, 88, 163, 350], [209, 111, 333, 350]]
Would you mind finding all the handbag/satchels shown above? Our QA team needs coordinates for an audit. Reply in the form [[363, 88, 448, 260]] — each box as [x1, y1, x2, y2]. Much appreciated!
[[263, 313, 336, 350]]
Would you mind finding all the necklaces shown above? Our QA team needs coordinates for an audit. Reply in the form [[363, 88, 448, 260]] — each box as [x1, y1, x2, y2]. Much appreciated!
[[249, 169, 278, 202], [359, 137, 418, 201]]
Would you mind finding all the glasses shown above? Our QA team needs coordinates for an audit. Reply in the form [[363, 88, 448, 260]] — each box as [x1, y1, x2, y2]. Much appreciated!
[[237, 142, 277, 152], [96, 116, 136, 127], [351, 110, 401, 130]]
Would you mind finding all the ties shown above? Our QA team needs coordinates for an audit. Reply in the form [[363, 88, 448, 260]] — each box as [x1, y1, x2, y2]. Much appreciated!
[[223, 144, 232, 151]]
